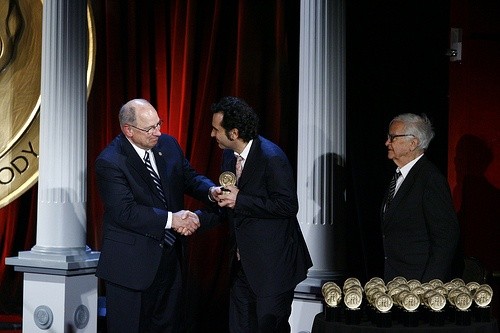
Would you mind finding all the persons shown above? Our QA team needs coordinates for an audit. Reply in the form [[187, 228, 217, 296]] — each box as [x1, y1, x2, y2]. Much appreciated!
[[95, 99, 222, 333], [381, 113, 460, 282], [174, 98, 313, 333]]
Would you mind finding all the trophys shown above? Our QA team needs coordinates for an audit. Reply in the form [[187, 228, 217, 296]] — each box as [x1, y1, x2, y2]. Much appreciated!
[[219, 171, 238, 202], [322, 276, 493, 327]]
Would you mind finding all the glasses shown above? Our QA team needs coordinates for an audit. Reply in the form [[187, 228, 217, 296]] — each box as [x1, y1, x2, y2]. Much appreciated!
[[387, 134, 415, 142], [126, 120, 163, 135]]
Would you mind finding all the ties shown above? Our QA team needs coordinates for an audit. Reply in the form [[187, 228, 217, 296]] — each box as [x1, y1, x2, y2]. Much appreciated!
[[143, 151, 176, 245], [384, 172, 402, 214], [236, 156, 242, 182]]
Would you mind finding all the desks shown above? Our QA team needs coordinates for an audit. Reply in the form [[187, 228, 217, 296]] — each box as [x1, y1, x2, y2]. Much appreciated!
[[312, 312, 500, 333]]
